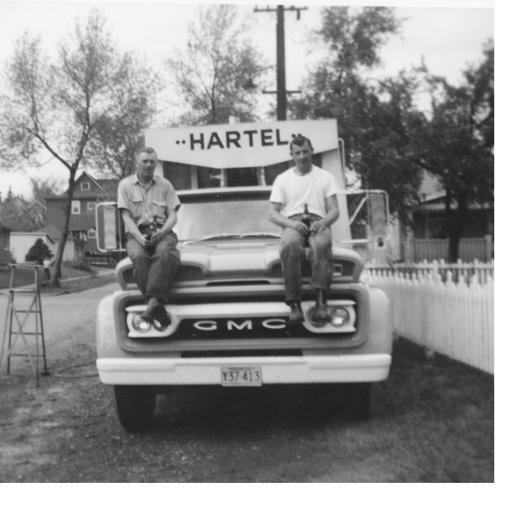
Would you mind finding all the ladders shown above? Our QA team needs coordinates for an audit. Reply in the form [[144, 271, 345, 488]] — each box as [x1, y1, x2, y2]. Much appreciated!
[[0, 264, 50, 388]]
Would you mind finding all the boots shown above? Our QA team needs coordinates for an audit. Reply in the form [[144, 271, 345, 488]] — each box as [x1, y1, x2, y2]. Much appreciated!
[[289, 302, 304, 324], [313, 289, 331, 322]]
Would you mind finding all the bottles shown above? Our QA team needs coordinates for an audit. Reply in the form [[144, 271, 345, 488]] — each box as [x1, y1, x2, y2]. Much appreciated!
[[144, 215, 158, 251], [300, 201, 312, 247]]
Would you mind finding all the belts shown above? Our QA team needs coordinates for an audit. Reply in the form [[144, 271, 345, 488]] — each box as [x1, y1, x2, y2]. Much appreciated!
[[139, 224, 162, 229]]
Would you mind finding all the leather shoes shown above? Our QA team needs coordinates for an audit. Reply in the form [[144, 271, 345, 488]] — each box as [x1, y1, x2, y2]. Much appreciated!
[[156, 308, 172, 326], [141, 298, 164, 321]]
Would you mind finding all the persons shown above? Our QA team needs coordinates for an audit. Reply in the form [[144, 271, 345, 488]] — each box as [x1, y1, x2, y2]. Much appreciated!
[[117, 145, 182, 323], [268, 132, 342, 325], [42, 255, 55, 280]]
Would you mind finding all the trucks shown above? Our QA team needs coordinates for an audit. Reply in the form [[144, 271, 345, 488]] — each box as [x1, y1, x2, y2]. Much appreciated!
[[95, 119, 394, 432]]
